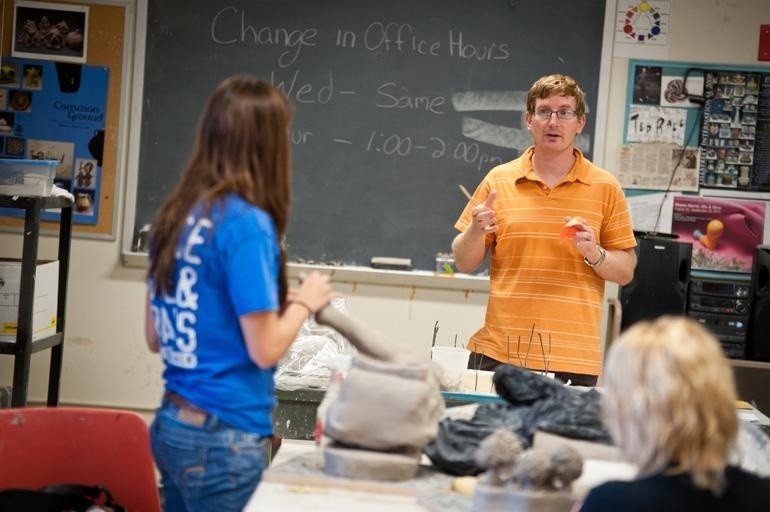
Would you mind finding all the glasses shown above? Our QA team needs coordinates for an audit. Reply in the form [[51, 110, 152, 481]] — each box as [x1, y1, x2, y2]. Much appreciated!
[[536, 108, 576, 119]]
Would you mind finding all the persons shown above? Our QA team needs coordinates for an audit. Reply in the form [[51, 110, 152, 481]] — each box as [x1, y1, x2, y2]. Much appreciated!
[[144, 76, 350, 512], [449, 73, 639, 385], [292, 270, 432, 366], [578, 315, 770, 512]]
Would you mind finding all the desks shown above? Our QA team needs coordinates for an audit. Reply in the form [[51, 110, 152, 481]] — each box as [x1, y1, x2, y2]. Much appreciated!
[[241, 433, 770, 512]]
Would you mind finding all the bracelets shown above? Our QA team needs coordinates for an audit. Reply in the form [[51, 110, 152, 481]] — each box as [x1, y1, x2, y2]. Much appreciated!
[[284, 297, 320, 317]]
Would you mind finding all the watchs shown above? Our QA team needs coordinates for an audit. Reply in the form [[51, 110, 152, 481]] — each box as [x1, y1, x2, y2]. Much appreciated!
[[584, 249, 609, 271]]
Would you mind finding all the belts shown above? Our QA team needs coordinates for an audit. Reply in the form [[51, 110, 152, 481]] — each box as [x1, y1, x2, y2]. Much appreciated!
[[167, 391, 210, 414]]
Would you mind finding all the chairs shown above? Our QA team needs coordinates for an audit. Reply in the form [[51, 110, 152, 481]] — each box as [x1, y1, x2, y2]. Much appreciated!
[[2, 404, 162, 512]]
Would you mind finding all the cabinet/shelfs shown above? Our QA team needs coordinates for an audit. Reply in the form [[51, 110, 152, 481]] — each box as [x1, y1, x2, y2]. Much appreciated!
[[0, 181, 78, 407]]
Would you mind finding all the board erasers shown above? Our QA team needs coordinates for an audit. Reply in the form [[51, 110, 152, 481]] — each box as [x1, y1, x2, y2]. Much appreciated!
[[370, 257, 413, 271]]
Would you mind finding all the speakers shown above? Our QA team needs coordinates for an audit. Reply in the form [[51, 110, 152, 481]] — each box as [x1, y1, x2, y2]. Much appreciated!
[[746, 245, 770, 362], [618, 236, 694, 336]]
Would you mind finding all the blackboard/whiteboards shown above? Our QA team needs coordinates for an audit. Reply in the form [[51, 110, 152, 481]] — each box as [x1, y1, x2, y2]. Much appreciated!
[[132, 0, 606, 276]]
